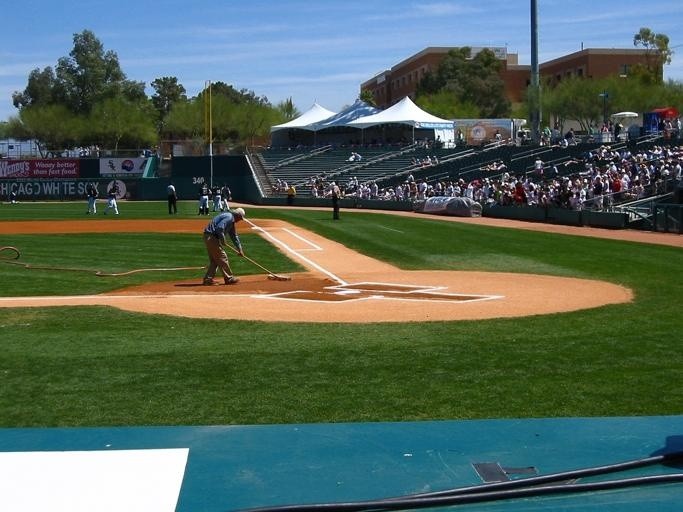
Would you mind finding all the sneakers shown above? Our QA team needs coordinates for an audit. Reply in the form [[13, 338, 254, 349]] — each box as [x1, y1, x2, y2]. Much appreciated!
[[202, 277, 241, 285]]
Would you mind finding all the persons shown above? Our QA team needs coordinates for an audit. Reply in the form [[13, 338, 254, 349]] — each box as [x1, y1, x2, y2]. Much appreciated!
[[221, 183, 231, 212], [330, 181, 341, 220], [74, 144, 100, 157], [10, 189, 17, 204], [198, 182, 222, 216], [86, 183, 98, 215], [202, 206, 246, 286], [104, 185, 119, 215], [167, 180, 178, 214]]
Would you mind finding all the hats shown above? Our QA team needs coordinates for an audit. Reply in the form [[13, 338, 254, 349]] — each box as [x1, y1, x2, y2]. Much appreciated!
[[235, 208, 244, 220]]
